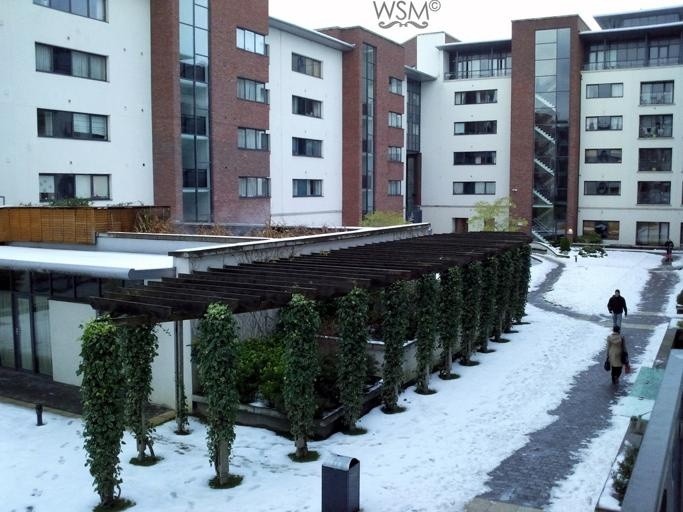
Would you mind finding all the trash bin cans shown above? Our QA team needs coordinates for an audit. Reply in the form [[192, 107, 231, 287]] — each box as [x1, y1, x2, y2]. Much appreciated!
[[322, 453, 360, 512]]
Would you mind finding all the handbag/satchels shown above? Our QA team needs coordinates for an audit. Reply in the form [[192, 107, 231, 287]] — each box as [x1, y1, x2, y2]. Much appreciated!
[[620, 336, 628, 364], [604, 359, 610, 371]]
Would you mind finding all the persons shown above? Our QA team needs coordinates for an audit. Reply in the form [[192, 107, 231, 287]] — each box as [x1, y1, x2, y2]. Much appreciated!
[[664, 238, 674, 262], [650, 122, 660, 137], [606, 326, 629, 385], [607, 290, 627, 330]]
